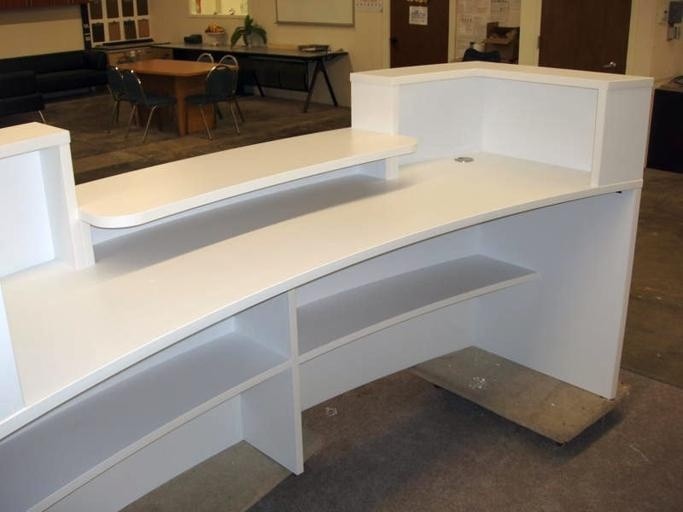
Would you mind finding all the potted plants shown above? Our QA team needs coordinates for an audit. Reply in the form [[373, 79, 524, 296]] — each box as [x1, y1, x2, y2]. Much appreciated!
[[230, 13, 268, 50]]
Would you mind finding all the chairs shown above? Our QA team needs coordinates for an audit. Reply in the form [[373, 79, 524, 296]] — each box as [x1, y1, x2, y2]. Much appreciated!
[[0, 70, 48, 123], [105, 53, 245, 143]]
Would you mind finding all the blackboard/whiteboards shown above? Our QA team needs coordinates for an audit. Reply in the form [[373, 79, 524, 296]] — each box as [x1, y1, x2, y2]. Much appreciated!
[[275, 0, 355, 27]]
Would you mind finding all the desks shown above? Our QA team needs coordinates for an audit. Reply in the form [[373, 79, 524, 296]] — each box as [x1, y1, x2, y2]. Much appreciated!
[[151, 42, 348, 114], [94, 40, 170, 66]]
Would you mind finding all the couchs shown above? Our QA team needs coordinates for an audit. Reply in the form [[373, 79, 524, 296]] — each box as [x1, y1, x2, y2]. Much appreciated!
[[0, 49, 110, 103]]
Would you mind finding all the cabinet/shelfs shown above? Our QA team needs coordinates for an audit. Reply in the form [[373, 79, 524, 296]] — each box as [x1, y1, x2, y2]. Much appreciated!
[[646, 80, 683, 173]]
